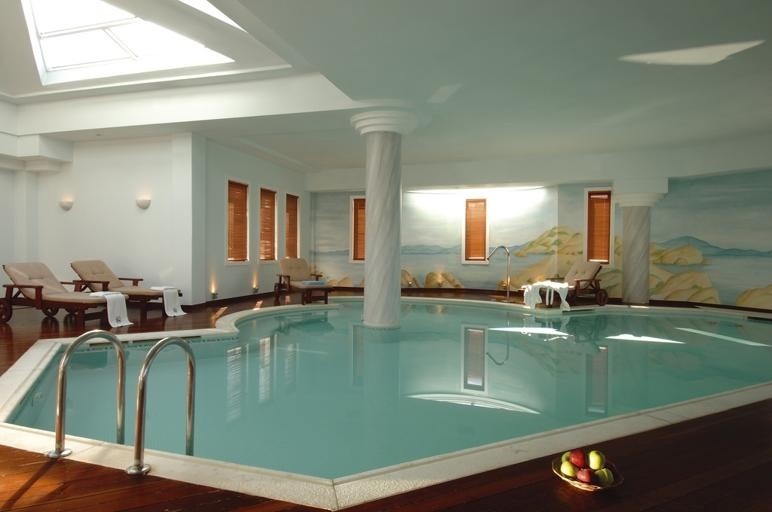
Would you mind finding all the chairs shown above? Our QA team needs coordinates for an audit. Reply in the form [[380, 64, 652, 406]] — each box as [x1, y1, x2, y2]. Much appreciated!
[[269, 311, 335, 346], [0, 257, 185, 327], [534, 313, 609, 354], [272, 257, 335, 306], [518, 258, 609, 310]]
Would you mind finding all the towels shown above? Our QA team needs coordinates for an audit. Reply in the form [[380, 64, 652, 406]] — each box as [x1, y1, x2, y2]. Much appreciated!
[[522, 279, 572, 311]]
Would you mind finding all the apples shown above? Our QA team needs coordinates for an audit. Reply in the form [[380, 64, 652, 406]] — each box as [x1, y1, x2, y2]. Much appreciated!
[[561, 449, 613, 486]]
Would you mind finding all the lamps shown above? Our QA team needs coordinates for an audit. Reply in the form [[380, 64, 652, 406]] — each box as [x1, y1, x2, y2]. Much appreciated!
[[210, 286, 260, 300], [58, 197, 152, 211]]
